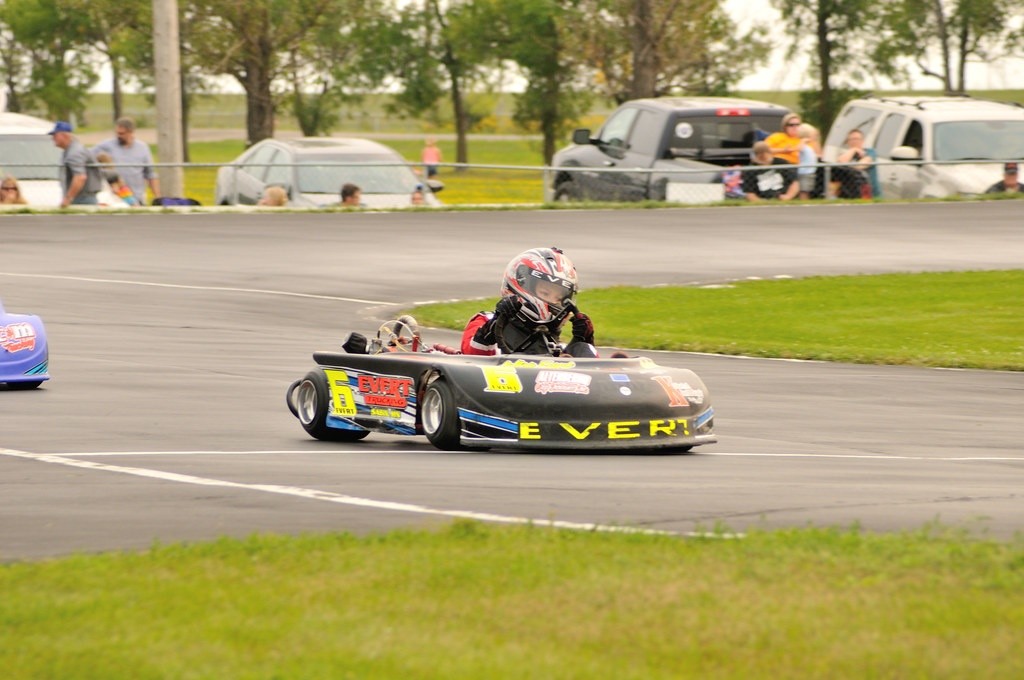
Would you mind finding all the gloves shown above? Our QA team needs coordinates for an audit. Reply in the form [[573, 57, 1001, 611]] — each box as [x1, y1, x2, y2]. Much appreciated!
[[483, 297, 522, 341], [570, 313, 594, 344]]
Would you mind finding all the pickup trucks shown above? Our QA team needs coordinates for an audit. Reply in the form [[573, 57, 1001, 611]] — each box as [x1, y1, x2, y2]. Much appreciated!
[[549, 97, 870, 203]]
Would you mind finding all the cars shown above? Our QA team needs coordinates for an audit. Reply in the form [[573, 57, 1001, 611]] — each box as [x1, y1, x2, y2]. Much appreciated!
[[0, 111, 130, 208], [218, 137, 444, 210]]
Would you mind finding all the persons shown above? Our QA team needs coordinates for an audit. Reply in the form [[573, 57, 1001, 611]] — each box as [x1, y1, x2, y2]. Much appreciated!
[[739, 112, 881, 200], [421, 137, 444, 176], [257, 186, 289, 207], [460, 245, 629, 358], [0, 176, 30, 203], [47, 121, 103, 207], [341, 183, 362, 207], [986, 162, 1024, 193], [412, 191, 424, 204], [90, 118, 162, 205]]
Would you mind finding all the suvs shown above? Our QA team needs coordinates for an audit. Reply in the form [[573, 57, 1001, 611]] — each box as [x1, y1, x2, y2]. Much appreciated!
[[825, 93, 1024, 198]]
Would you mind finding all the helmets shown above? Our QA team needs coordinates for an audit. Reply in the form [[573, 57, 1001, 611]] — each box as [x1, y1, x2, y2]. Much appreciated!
[[501, 247, 578, 335]]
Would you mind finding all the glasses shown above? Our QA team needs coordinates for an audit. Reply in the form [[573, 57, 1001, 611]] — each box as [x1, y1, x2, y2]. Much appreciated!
[[785, 124, 801, 127]]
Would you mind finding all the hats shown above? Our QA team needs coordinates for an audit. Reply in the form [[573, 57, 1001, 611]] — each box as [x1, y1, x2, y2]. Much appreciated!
[[752, 141, 771, 153], [1005, 163, 1018, 173], [48, 121, 72, 135]]
